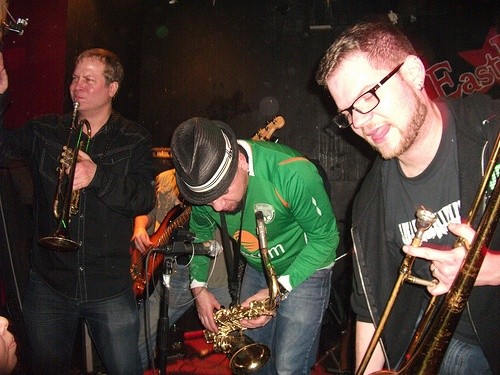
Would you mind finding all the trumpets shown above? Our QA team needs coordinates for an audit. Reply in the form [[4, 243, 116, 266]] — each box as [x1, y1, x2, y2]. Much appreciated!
[[36, 102, 91, 252]]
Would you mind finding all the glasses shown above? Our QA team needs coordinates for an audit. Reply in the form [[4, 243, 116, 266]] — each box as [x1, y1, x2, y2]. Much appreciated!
[[332, 61, 404, 129]]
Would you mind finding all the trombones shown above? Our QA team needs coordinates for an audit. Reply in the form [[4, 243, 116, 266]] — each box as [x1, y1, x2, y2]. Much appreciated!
[[352, 133, 499, 375]]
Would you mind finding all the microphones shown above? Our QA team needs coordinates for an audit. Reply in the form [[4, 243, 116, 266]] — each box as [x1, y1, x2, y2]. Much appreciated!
[[152, 240, 223, 257]]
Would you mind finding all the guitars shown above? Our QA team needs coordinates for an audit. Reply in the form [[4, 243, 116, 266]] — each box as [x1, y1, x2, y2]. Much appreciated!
[[131, 114, 285, 294]]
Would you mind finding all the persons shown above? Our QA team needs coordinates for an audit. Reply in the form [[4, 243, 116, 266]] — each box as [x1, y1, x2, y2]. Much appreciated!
[[0, 315, 17, 375], [316, 20, 500, 375], [132, 167, 194, 370], [171, 116, 340, 374], [0, 49, 157, 375]]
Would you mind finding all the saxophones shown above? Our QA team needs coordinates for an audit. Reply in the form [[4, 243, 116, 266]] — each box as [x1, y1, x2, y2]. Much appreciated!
[[202, 210, 285, 375]]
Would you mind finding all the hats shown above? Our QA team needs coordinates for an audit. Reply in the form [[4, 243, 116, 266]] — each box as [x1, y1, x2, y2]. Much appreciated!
[[170, 117, 239, 206]]
